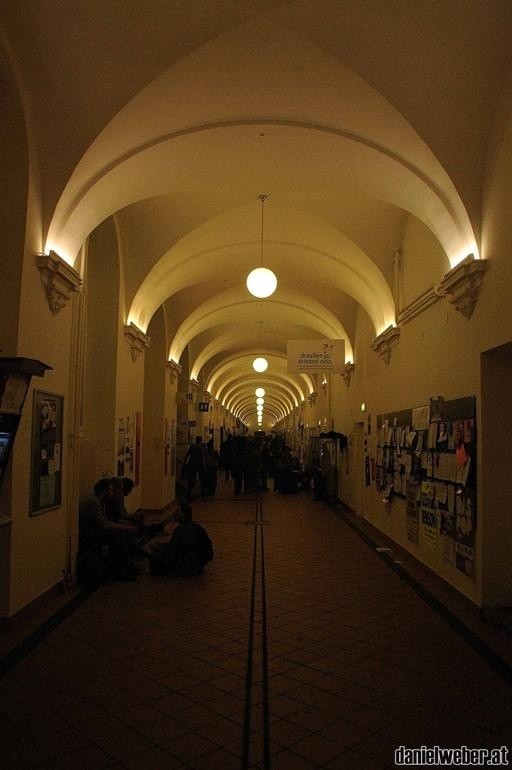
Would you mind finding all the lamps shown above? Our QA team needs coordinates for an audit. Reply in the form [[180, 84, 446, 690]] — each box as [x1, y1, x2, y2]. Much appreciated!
[[252, 321, 268, 426], [246, 195, 277, 299]]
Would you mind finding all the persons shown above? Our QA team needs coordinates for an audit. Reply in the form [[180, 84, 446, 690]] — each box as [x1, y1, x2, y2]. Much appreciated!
[[110, 475, 136, 578], [180, 424, 311, 498], [78, 480, 141, 581], [146, 500, 212, 578], [116, 476, 147, 543]]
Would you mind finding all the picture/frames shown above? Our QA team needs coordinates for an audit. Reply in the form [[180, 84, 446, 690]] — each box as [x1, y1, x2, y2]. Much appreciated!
[[28, 388, 64, 518]]
